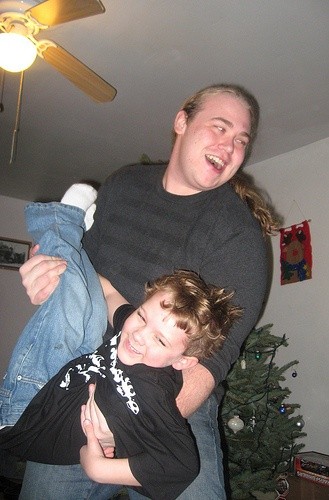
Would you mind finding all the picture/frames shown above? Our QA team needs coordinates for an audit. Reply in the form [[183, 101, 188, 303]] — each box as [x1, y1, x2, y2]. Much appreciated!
[[0, 236, 32, 271]]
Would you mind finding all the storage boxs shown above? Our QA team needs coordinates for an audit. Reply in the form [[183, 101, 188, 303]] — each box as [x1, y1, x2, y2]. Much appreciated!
[[294, 450, 329, 488]]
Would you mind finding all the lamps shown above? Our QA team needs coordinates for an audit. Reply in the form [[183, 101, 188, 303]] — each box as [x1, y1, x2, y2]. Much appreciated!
[[0, 22, 37, 73]]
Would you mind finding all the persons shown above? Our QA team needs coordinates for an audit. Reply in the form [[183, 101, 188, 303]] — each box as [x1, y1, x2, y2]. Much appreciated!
[[17, 84, 276, 500], [0, 184, 242, 500]]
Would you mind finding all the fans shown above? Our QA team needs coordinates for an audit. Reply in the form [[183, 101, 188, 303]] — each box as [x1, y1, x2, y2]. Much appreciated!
[[0, 0, 118, 104]]
